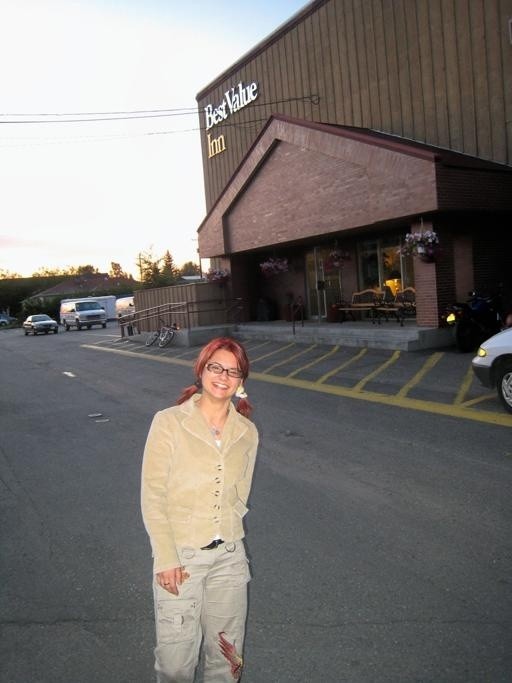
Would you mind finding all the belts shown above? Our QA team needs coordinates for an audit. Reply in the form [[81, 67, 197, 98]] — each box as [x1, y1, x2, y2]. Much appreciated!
[[201, 539, 223, 549]]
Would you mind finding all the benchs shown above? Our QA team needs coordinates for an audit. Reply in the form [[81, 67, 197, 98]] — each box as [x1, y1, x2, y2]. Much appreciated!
[[335, 286, 416, 326]]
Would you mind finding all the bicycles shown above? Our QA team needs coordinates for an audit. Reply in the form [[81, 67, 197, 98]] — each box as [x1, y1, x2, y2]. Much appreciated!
[[145, 324, 176, 347]]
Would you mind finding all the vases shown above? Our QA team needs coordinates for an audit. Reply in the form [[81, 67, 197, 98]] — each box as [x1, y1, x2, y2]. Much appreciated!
[[215, 279, 228, 284], [273, 270, 280, 274], [417, 243, 425, 254], [333, 261, 341, 267]]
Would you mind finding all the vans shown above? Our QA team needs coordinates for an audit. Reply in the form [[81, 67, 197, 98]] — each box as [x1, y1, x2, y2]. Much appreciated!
[[60, 300, 107, 330]]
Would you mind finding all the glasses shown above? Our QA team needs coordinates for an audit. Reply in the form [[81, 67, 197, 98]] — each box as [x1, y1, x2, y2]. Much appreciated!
[[206, 362, 245, 379]]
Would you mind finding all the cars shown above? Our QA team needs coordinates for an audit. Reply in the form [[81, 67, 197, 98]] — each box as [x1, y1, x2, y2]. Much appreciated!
[[471, 326, 512, 414], [0, 313, 18, 327], [23, 313, 58, 335]]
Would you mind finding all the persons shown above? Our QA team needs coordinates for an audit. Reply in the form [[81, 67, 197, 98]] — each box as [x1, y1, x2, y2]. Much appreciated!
[[140, 336, 262, 682]]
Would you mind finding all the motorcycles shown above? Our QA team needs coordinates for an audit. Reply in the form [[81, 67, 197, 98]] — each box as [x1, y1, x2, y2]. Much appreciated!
[[440, 284, 503, 351]]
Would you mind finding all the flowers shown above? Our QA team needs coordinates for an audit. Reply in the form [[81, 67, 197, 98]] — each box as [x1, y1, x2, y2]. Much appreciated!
[[397, 230, 444, 260], [260, 257, 288, 276], [205, 267, 232, 284], [325, 250, 352, 268]]
